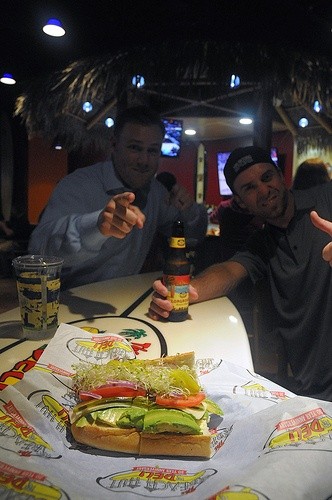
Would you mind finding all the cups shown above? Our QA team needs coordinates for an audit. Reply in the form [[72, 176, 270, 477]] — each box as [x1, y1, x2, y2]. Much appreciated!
[[12, 254, 65, 340]]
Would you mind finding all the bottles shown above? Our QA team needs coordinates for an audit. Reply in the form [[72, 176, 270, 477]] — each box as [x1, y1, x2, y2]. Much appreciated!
[[162, 221, 190, 321]]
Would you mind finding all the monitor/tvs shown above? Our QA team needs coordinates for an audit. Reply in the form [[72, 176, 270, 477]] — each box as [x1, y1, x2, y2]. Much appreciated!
[[216, 147, 278, 197], [161, 119, 183, 158]]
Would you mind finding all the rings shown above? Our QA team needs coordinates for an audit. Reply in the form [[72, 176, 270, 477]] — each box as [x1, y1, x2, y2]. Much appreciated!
[[177, 199, 184, 206]]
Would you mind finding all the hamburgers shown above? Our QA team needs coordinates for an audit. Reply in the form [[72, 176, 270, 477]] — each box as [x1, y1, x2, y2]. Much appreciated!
[[70, 351, 212, 459]]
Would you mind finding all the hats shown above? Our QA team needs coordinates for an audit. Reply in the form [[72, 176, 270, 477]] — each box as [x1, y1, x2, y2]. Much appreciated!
[[223, 146, 277, 193]]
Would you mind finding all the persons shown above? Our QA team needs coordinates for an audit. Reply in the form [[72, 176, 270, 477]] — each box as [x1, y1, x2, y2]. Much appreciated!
[[0, 205, 30, 268], [149, 147, 332, 402], [27, 105, 208, 291], [293, 161, 331, 189], [66, 130, 251, 259]]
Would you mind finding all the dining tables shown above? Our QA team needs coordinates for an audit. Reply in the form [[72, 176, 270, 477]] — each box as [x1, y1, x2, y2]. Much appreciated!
[[0, 262, 332, 500]]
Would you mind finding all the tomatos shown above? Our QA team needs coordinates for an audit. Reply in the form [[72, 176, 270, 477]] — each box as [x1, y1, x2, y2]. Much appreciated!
[[155, 392, 206, 408]]
